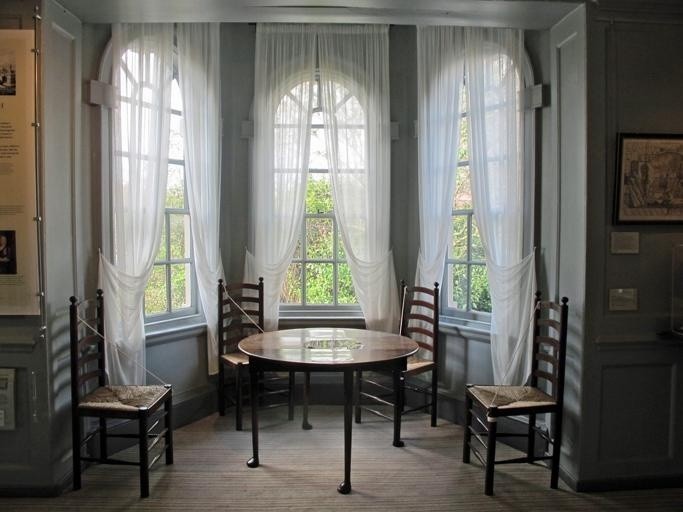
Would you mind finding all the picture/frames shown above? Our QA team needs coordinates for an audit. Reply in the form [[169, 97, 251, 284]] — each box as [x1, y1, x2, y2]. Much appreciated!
[[609, 130, 682, 228]]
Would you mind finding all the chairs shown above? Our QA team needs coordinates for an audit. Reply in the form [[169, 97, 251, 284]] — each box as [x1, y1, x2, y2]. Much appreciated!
[[215, 276, 299, 431], [66, 287, 174, 497], [454, 287, 570, 499], [353, 278, 442, 448]]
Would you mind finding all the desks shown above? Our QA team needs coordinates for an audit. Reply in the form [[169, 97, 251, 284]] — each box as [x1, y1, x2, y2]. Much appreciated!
[[237, 326, 423, 493]]
